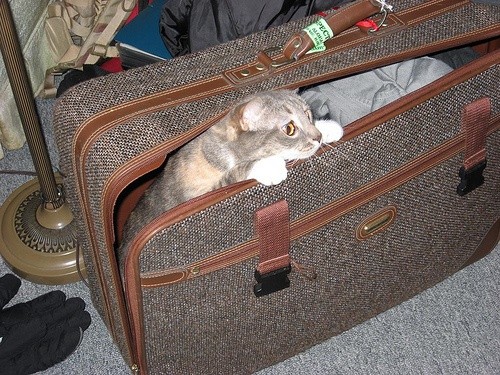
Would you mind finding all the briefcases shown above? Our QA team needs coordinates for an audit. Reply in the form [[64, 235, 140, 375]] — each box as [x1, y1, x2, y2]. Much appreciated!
[[51, 0, 500, 375]]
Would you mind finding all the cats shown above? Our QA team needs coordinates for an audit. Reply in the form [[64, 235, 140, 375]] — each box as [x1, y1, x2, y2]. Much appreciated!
[[116, 86, 355, 303]]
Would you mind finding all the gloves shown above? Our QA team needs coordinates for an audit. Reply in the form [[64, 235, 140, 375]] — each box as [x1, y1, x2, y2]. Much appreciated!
[[0, 274, 91, 375]]
